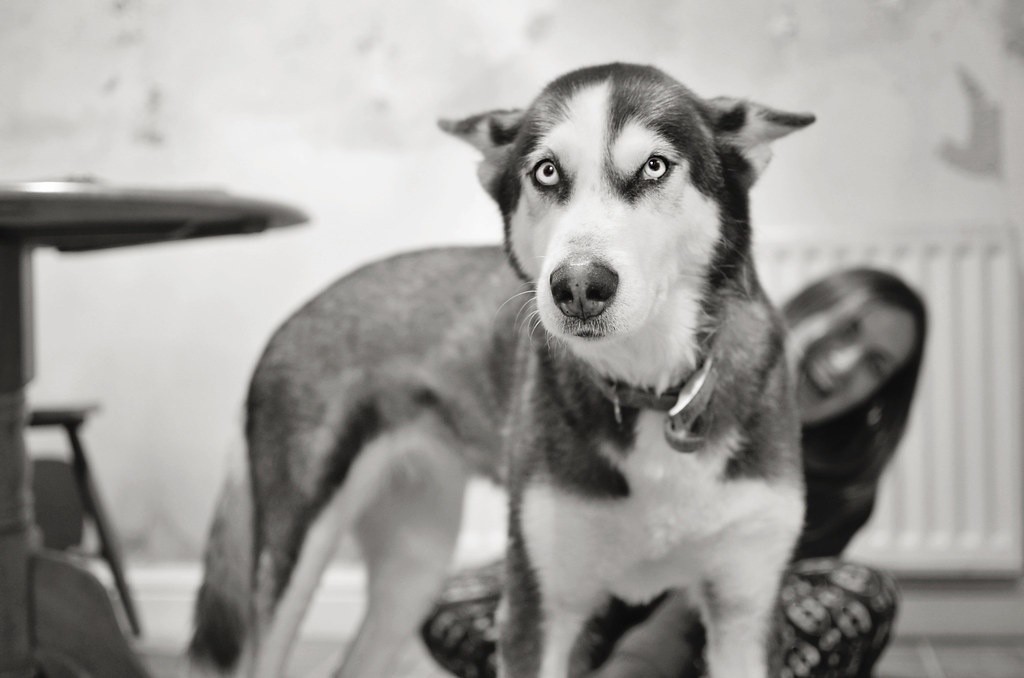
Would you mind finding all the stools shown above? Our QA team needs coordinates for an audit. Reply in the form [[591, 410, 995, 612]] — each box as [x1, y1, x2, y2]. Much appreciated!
[[24, 400, 148, 635]]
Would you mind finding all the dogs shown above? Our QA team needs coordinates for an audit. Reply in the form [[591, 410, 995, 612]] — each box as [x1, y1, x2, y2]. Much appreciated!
[[180, 63, 816, 678]]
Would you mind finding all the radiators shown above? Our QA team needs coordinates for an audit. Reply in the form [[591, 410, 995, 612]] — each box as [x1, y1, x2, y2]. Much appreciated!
[[754, 225, 1024, 574]]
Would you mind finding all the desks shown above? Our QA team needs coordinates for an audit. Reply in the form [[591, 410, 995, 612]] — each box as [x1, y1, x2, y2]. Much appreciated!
[[0, 184, 308, 676]]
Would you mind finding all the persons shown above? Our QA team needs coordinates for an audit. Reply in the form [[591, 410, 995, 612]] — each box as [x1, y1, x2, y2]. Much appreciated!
[[420, 265, 929, 678]]
